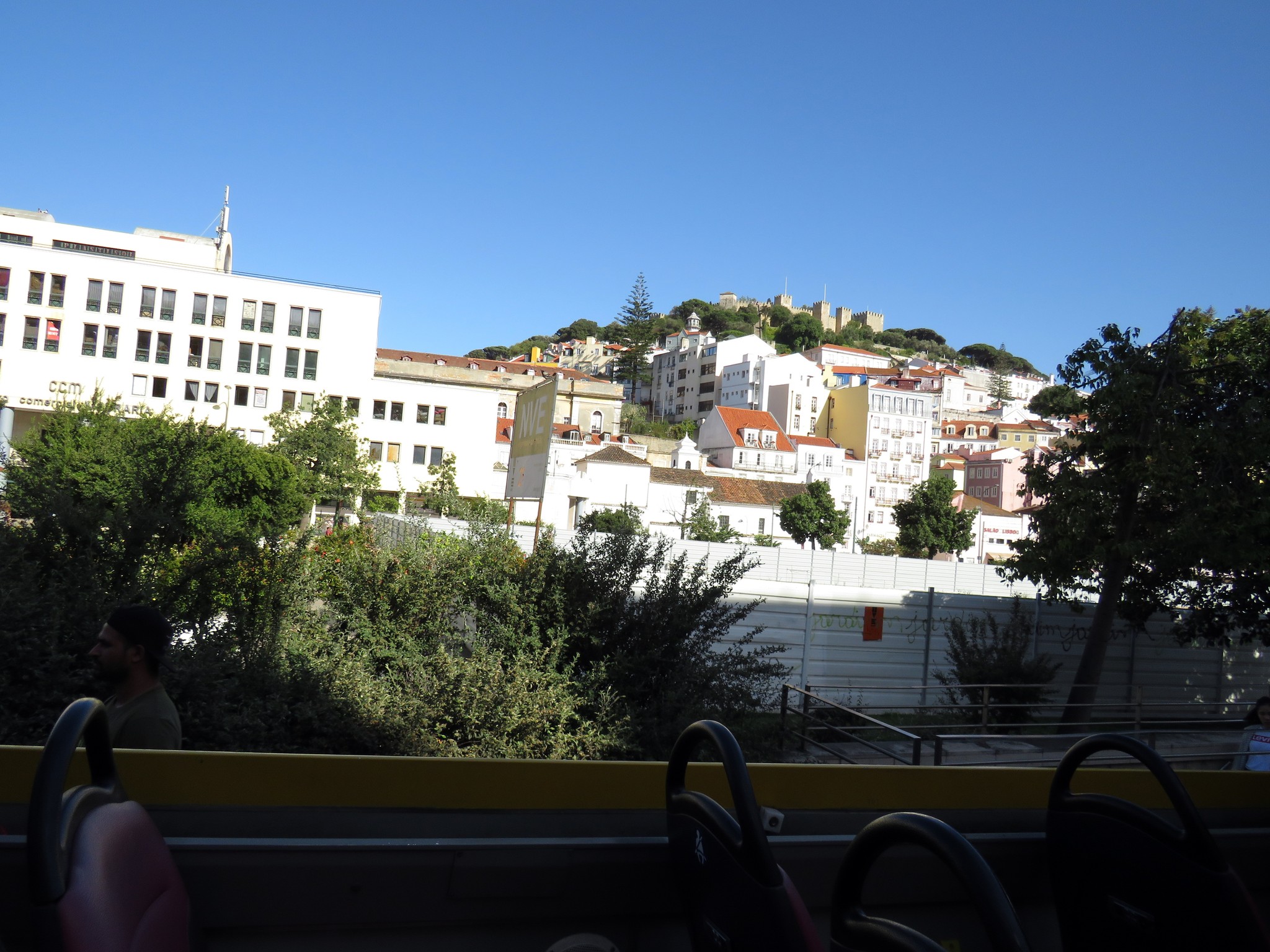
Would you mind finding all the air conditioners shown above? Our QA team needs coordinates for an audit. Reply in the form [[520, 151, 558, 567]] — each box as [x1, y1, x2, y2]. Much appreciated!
[[1010, 459, 1014, 463], [1006, 458, 1009, 463]]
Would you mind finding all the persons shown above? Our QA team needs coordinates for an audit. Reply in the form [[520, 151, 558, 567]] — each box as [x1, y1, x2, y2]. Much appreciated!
[[1232, 696, 1270, 771], [77, 603, 182, 751]]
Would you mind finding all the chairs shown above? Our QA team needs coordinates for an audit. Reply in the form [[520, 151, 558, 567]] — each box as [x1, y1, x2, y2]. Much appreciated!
[[31, 699, 195, 952], [665, 720, 826, 952], [829, 809, 1033, 951], [1046, 732, 1270, 952]]
